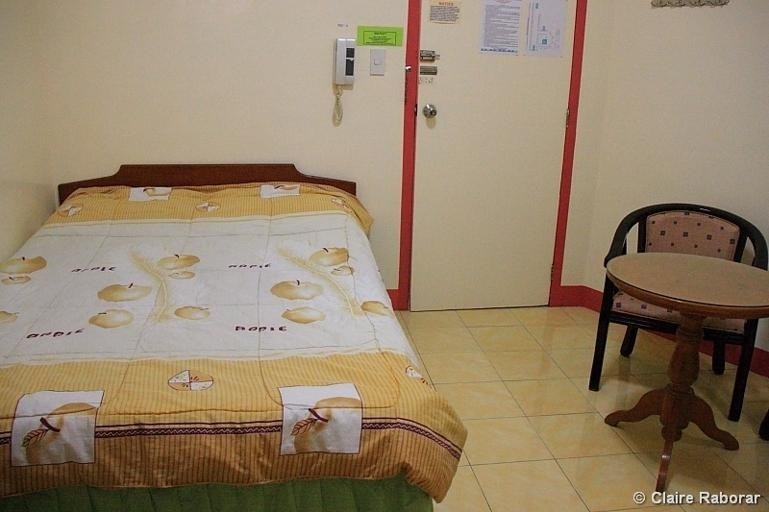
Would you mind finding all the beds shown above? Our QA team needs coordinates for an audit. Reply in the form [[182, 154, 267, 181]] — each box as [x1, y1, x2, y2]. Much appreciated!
[[0, 163, 470, 512]]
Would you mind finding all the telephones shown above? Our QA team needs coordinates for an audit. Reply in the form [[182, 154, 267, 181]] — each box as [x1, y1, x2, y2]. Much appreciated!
[[335, 38, 355, 85]]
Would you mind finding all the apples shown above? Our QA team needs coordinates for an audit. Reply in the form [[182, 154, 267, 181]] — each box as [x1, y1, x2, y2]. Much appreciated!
[[89, 253, 210, 329], [142, 187, 171, 197], [271, 246, 391, 325], [0, 255, 47, 322], [290, 397, 362, 453], [21, 402, 98, 466], [274, 184, 299, 190]]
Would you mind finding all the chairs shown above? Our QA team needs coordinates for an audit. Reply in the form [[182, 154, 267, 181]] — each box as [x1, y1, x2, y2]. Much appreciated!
[[589, 201, 769, 440]]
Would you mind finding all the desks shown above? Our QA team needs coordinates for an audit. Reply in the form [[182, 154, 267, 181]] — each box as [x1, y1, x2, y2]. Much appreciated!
[[604, 250, 769, 493]]
